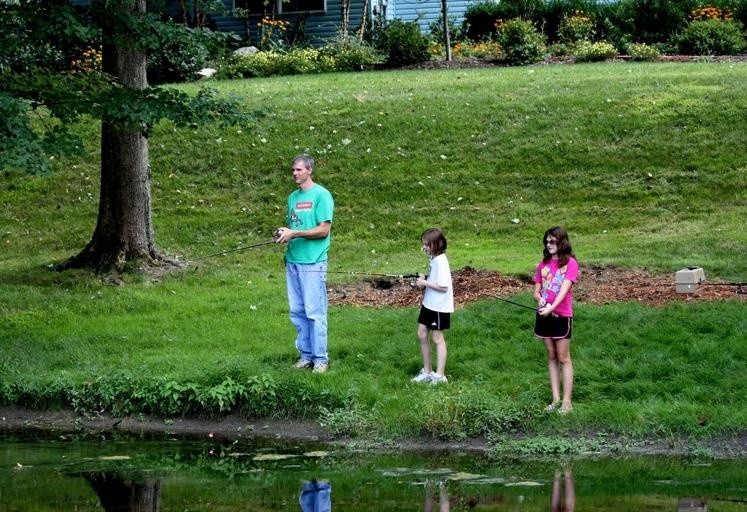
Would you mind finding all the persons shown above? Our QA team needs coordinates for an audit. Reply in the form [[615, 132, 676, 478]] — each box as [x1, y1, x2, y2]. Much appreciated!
[[299, 479, 332, 512], [551, 470, 575, 512], [533, 226, 579, 415], [410, 228, 455, 387], [424, 490, 450, 512], [272, 155, 334, 373]]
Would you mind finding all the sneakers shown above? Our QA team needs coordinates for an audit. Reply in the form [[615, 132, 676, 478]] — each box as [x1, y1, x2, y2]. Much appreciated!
[[292, 359, 312, 370], [298, 475, 330, 486], [428, 376, 448, 387], [410, 368, 435, 384], [543, 403, 572, 416], [312, 363, 328, 375]]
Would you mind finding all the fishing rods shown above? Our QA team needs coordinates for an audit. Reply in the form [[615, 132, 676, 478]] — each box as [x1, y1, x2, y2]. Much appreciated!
[[144, 238, 279, 271], [483, 290, 541, 313], [271, 266, 418, 280]]
